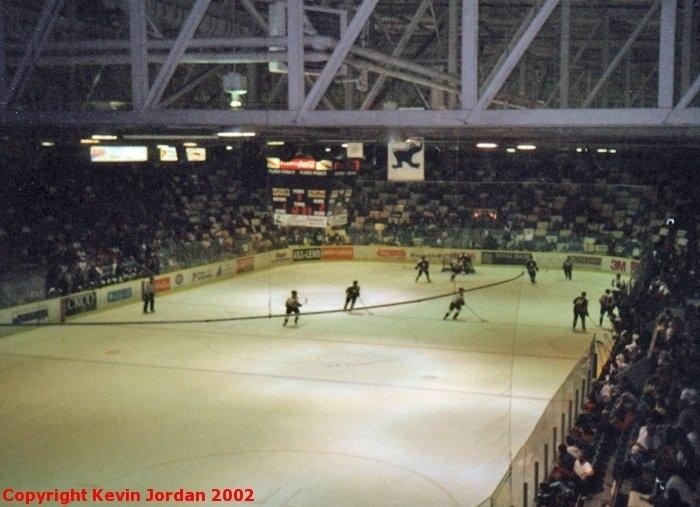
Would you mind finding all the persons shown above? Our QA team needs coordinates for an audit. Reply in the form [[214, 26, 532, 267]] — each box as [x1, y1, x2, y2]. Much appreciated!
[[348, 155, 535, 253], [343, 280, 361, 311], [142, 274, 157, 313], [415, 255, 432, 284], [535, 147, 699, 504], [526, 255, 539, 282], [2, 156, 346, 299], [450, 254, 472, 283], [283, 290, 301, 326], [443, 287, 465, 320]]
